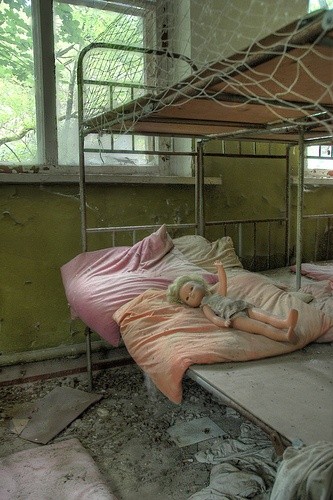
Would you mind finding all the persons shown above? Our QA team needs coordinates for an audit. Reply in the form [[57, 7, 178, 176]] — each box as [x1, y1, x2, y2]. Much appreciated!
[[166, 259, 299, 345]]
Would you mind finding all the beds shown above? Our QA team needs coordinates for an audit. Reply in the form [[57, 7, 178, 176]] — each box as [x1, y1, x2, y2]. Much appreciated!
[[74, 7, 333, 467]]
